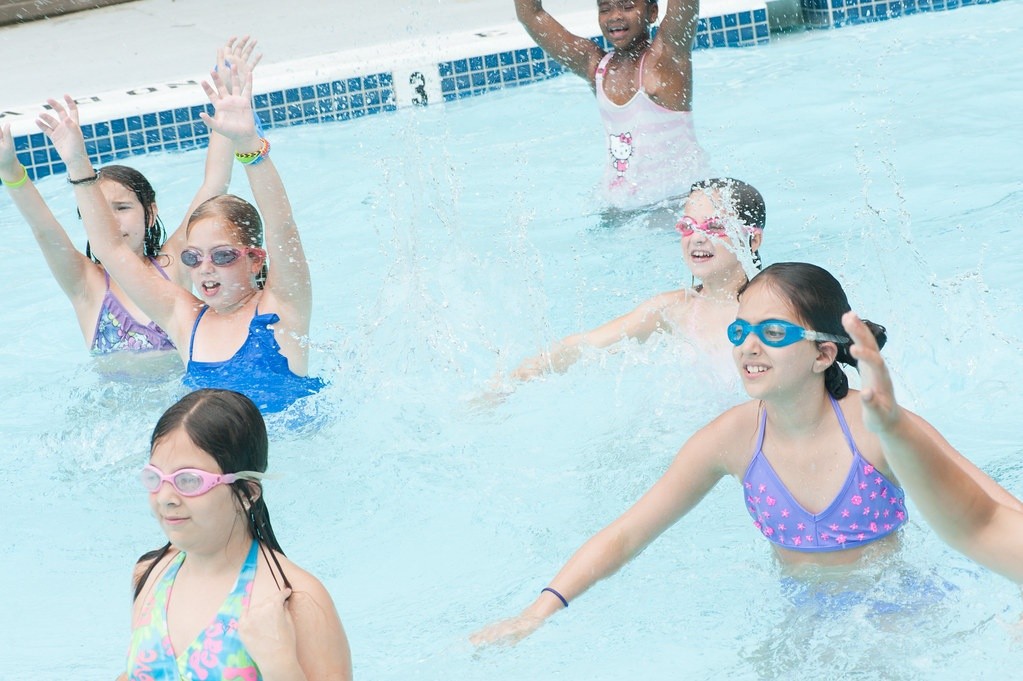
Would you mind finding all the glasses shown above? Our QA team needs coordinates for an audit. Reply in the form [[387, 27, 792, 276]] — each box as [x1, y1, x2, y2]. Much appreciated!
[[675, 216, 762, 238], [727, 319, 850, 348], [180, 244, 265, 268], [140, 465, 264, 496]]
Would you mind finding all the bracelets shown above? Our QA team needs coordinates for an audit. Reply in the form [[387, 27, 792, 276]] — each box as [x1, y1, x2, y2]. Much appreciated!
[[67, 169, 101, 187], [541, 588, 569, 609], [235, 138, 271, 166], [1, 164, 28, 188]]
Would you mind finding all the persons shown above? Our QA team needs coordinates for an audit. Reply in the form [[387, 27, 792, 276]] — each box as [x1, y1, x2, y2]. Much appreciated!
[[0, 35, 335, 441], [112, 388, 354, 680], [514, 0, 714, 241], [842, 309, 1022, 588], [467, 261, 1021, 652], [505, 176, 766, 386]]
